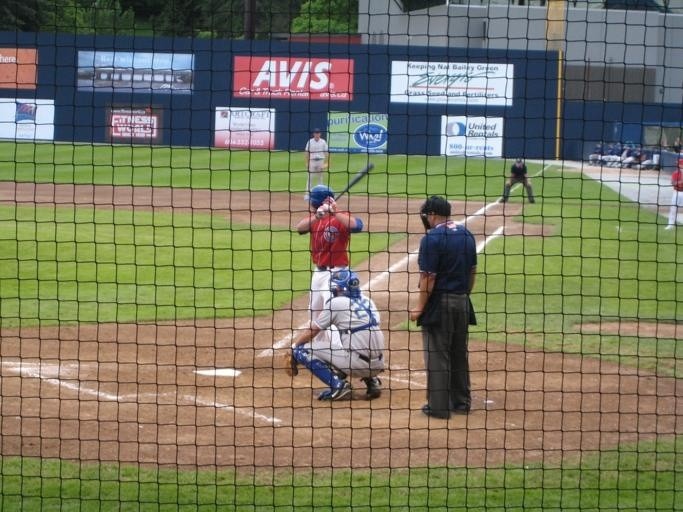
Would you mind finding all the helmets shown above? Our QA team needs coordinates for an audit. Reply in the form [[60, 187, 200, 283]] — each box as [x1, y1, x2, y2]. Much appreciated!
[[329, 269, 360, 298], [310, 184, 334, 208]]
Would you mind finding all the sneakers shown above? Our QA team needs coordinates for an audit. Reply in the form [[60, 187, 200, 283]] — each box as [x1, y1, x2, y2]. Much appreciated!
[[304, 195, 309, 200], [665, 224, 675, 230]]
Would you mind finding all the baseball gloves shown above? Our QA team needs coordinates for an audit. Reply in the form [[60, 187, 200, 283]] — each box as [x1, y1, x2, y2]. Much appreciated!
[[283, 346, 299, 377], [321, 164, 329, 170]]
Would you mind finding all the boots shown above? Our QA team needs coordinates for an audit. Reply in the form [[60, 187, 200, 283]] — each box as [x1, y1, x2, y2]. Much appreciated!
[[366, 376, 383, 393], [322, 380, 351, 398]]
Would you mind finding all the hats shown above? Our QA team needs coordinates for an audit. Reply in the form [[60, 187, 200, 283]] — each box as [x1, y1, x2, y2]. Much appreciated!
[[313, 128, 321, 132]]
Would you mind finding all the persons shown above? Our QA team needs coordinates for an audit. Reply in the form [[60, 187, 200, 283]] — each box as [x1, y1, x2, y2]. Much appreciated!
[[304, 128, 328, 200], [499, 157, 535, 203], [589, 141, 660, 171], [409, 195, 477, 419], [285, 270, 386, 402], [665, 158, 683, 230], [296, 184, 364, 345], [673, 136, 683, 153]]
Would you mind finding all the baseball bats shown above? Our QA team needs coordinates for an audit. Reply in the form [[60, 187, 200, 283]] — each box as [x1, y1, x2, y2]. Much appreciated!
[[318, 161, 374, 216]]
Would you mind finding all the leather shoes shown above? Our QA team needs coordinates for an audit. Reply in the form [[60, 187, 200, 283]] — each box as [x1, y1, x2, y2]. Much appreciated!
[[421, 400, 470, 418]]
